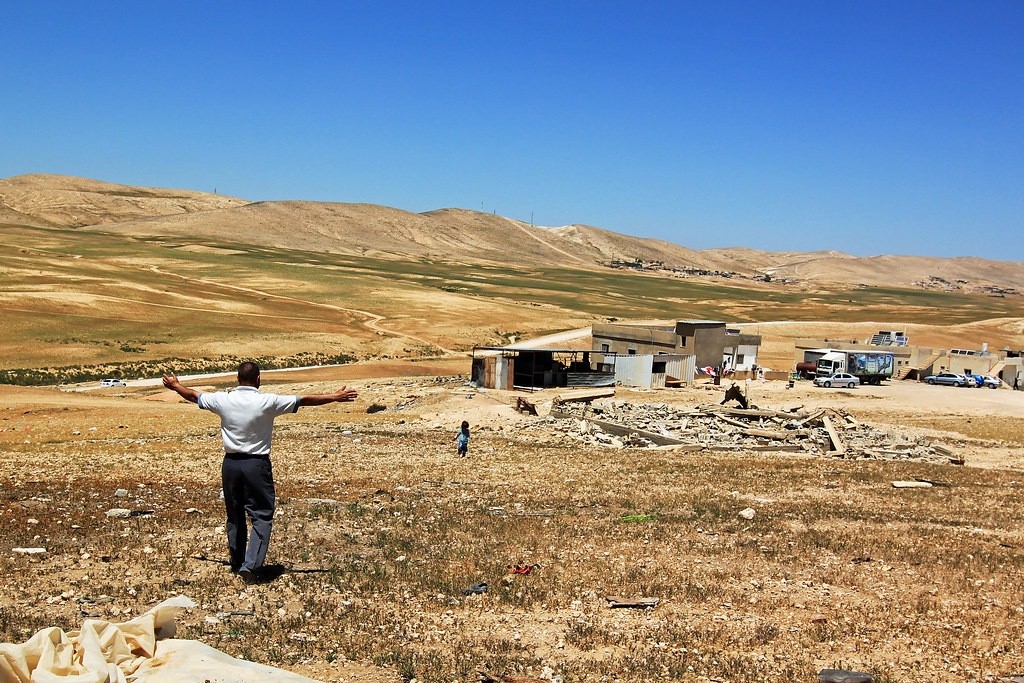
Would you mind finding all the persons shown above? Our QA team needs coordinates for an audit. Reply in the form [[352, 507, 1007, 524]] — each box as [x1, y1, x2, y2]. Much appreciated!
[[452, 421, 472, 457], [162, 362, 358, 585], [1014, 378, 1019, 390]]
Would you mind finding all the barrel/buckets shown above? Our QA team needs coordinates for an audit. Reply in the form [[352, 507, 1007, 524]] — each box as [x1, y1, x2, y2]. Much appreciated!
[[789, 380, 794, 387]]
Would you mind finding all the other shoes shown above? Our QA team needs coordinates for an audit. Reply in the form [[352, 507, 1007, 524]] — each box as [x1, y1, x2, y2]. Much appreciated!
[[237, 571, 260, 585]]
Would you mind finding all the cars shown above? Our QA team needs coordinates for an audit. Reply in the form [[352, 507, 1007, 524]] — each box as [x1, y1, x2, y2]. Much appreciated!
[[982, 375, 1003, 390], [813, 372, 861, 389], [925, 372, 968, 387], [959, 373, 985, 388], [100, 378, 127, 388]]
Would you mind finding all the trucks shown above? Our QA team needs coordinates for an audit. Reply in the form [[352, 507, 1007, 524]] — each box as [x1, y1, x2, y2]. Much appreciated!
[[815, 350, 895, 385]]
